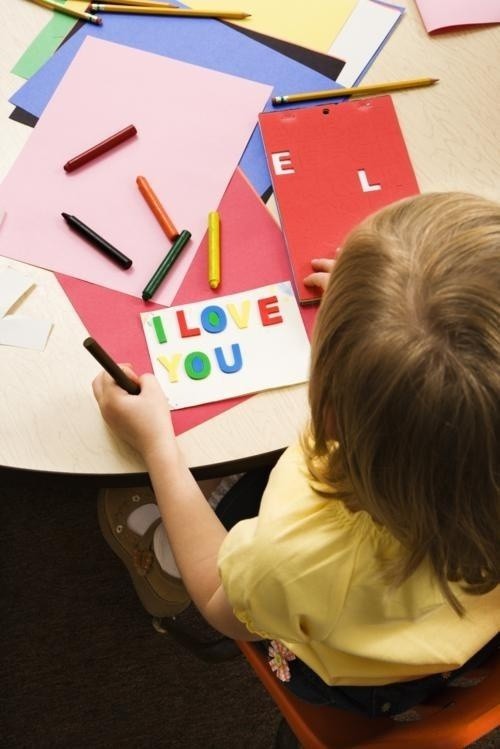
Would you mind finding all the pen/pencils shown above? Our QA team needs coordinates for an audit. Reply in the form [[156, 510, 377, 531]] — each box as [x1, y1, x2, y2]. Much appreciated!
[[24, 1, 251, 27], [63, 123, 137, 173], [271, 76, 441, 107], [208, 212, 221, 289], [136, 175, 179, 242], [61, 212, 132, 270], [83, 337, 139, 395], [142, 229, 192, 301]]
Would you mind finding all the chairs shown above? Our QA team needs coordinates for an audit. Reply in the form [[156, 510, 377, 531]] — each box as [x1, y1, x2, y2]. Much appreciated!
[[235, 642, 499, 749]]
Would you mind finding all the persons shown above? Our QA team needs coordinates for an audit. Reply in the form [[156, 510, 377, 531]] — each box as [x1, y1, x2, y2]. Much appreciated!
[[94, 188, 500, 719]]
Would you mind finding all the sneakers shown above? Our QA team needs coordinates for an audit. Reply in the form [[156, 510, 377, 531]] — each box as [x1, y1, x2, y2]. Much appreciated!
[[97, 486, 191, 618]]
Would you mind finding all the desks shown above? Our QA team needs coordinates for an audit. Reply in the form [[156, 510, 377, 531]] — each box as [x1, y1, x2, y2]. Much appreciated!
[[3, 4, 500, 489]]
